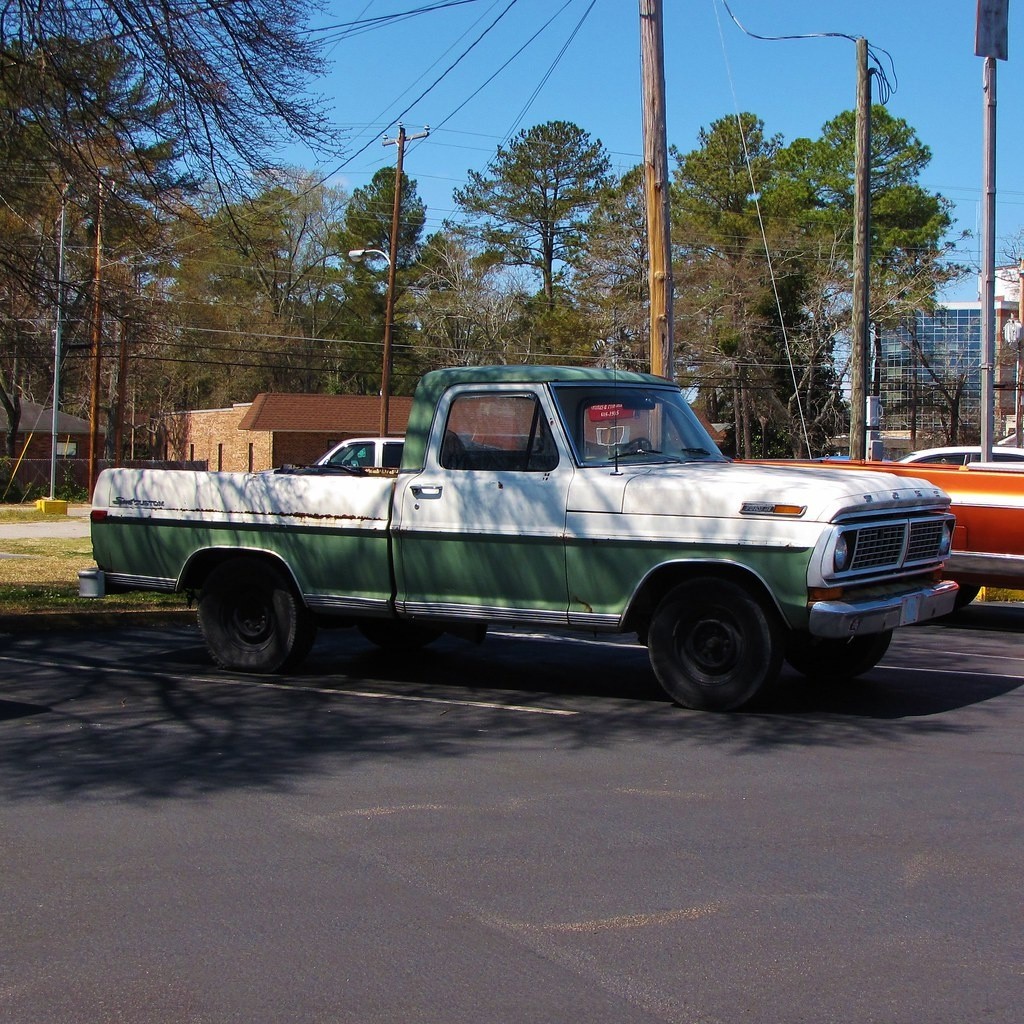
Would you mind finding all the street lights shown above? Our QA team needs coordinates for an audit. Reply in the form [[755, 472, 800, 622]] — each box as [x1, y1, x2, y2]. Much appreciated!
[[347, 248, 394, 438]]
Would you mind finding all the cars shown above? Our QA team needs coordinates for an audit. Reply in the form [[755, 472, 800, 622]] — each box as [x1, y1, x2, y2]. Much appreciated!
[[898, 446, 1024, 470], [310, 436, 411, 475]]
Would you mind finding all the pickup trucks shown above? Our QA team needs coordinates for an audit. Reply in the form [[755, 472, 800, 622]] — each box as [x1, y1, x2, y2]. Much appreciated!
[[730, 454, 1024, 616], [95, 363, 986, 710]]
